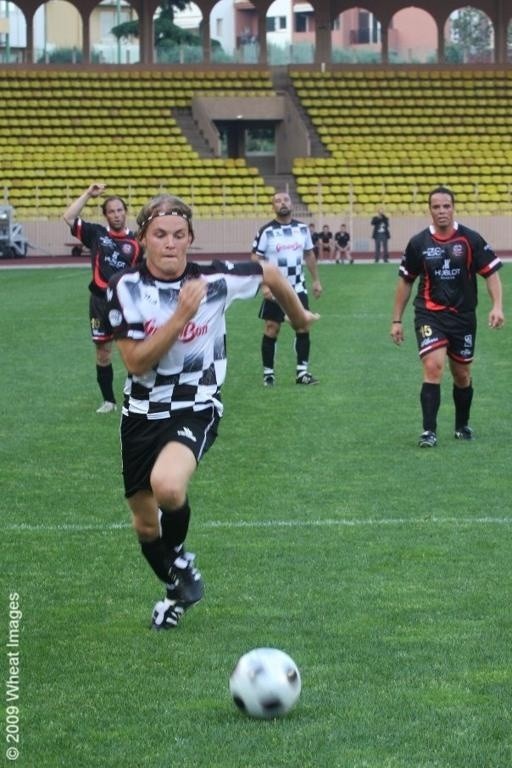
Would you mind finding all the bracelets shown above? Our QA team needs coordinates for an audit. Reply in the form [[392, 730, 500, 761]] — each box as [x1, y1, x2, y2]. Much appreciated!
[[391, 319, 402, 325]]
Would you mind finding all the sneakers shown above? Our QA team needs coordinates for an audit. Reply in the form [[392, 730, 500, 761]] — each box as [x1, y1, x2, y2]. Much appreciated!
[[151, 550, 205, 631], [296, 371, 320, 385], [96, 401, 118, 413], [263, 373, 277, 386], [416, 430, 439, 447], [455, 427, 475, 442]]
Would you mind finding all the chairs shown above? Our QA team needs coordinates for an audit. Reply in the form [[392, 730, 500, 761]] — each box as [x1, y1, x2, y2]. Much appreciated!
[[288, 68, 512, 218]]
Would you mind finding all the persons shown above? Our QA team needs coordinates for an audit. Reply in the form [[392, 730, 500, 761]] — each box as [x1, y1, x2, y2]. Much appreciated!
[[318, 224, 333, 260], [334, 225, 355, 264], [60, 182, 147, 414], [101, 193, 322, 627], [307, 223, 319, 260], [389, 187, 505, 449], [368, 208, 391, 262], [250, 193, 322, 388]]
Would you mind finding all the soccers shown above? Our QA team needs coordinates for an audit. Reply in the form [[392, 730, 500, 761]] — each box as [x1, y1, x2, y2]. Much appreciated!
[[229, 649, 301, 718]]
